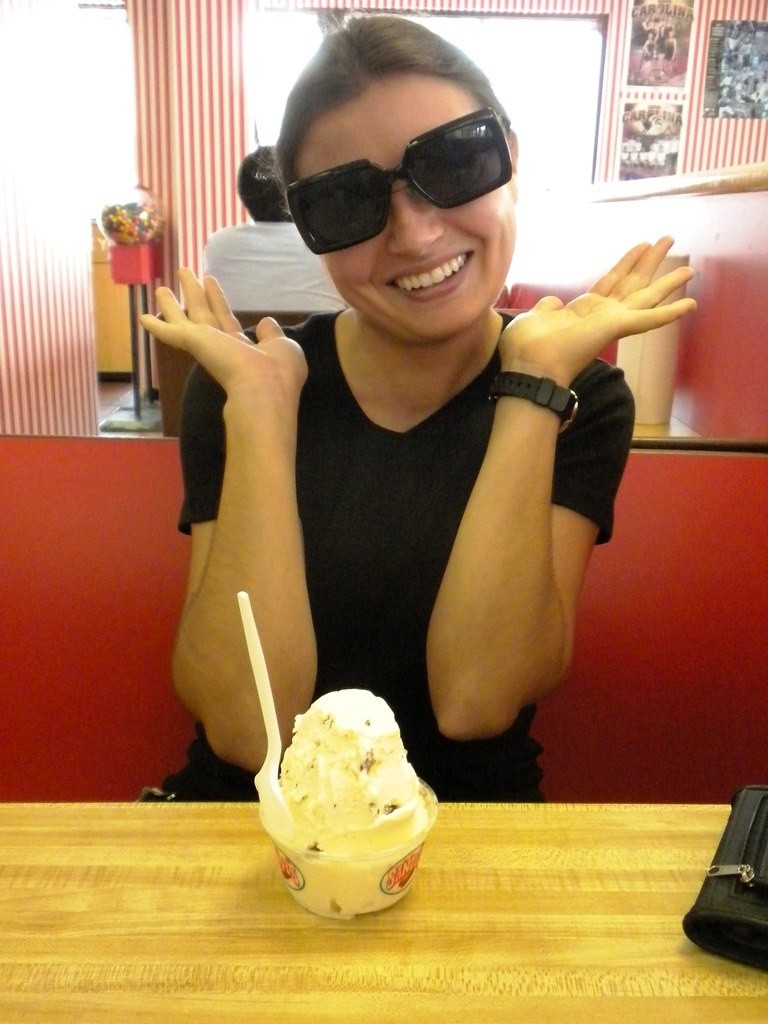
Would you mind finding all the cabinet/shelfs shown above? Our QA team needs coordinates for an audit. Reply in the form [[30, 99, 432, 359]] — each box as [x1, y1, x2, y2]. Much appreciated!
[[90, 217, 134, 374]]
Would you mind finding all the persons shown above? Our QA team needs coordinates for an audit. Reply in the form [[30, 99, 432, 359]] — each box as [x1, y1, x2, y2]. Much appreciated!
[[201, 145, 350, 315], [138, 12, 699, 804], [621, 13, 767, 167]]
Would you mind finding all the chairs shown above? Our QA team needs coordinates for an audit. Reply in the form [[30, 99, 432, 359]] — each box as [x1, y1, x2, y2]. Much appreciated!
[[154, 308, 334, 437], [512, 283, 618, 369]]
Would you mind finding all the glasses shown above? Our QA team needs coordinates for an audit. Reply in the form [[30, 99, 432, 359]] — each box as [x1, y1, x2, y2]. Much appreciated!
[[282, 106, 513, 255]]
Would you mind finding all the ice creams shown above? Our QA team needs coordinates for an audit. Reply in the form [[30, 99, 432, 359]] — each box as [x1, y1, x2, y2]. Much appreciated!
[[261, 689, 440, 920]]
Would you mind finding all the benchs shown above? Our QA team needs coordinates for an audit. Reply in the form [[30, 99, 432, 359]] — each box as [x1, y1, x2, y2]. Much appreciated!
[[1, 432, 768, 802]]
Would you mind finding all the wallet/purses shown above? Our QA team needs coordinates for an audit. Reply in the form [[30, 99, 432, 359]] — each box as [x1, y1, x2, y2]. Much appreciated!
[[682, 785, 768, 967]]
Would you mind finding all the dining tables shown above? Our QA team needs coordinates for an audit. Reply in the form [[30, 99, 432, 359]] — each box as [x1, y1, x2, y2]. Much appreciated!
[[1, 801, 768, 1024]]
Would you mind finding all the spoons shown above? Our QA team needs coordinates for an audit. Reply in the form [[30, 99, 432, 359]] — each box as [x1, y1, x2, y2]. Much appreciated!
[[236, 588, 295, 842]]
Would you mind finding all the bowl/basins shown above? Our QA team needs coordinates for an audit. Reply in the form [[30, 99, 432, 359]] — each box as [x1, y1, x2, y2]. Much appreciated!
[[256, 776, 439, 921]]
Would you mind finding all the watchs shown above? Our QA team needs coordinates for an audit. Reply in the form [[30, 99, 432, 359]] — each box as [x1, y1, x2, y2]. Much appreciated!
[[490, 369, 579, 435]]
